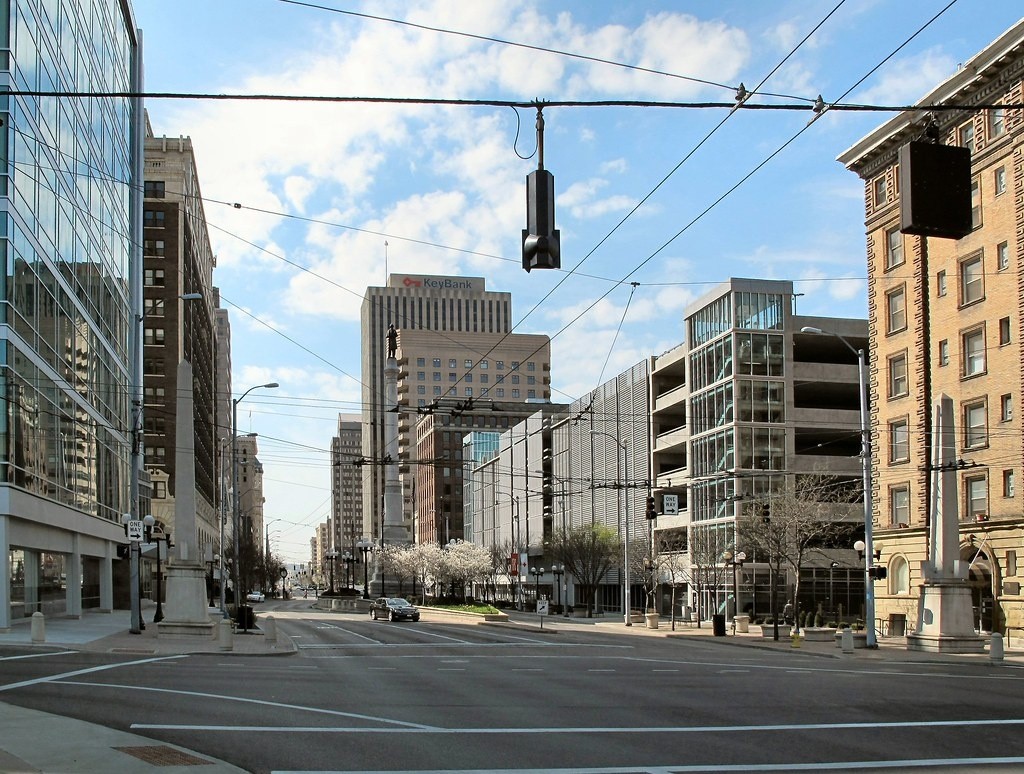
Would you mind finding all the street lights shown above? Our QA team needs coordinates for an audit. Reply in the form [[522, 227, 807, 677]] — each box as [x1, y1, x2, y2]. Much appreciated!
[[444, 538, 457, 604], [325, 548, 339, 595], [495, 491, 522, 611], [534, 470, 569, 618], [220, 432, 263, 612], [341, 551, 353, 593], [551, 564, 565, 614], [204, 554, 220, 607], [722, 551, 746, 637], [265, 518, 280, 595], [643, 557, 661, 613], [799, 325, 878, 649], [429, 509, 450, 544], [589, 429, 632, 626], [233, 382, 280, 621], [356, 535, 374, 599], [121, 512, 156, 631], [224, 558, 233, 604], [127, 292, 205, 634], [531, 567, 545, 599]]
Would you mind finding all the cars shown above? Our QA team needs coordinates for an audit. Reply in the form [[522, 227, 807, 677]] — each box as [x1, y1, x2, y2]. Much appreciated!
[[369, 596, 421, 622], [246, 590, 265, 603]]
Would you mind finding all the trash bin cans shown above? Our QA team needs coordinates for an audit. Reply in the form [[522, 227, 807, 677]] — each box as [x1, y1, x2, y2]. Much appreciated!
[[713, 615, 725, 636], [237, 605, 253, 629]]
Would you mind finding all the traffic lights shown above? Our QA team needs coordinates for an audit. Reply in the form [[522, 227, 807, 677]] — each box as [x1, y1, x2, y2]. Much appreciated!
[[646, 497, 657, 519], [763, 503, 771, 523]]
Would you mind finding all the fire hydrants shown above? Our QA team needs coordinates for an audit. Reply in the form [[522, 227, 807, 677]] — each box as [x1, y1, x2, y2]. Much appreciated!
[[790, 628, 801, 648]]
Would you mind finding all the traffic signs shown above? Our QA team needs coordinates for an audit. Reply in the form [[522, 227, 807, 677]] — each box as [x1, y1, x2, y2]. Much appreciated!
[[663, 494, 679, 515], [127, 520, 144, 542]]
